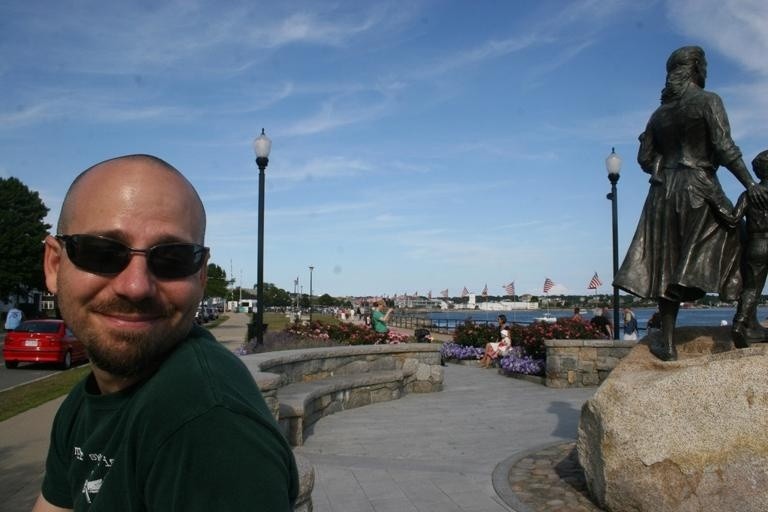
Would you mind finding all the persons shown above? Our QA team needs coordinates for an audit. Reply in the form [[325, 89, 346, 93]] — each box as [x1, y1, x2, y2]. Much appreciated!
[[479, 316, 513, 369], [706, 147, 768, 349], [31, 153, 299, 512], [4, 303, 28, 332], [611, 45, 768, 360], [370, 300, 394, 345], [573, 306, 662, 341], [323, 304, 363, 322]]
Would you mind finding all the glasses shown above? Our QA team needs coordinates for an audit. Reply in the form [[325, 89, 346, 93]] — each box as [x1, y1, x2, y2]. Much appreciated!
[[55, 234, 210, 280]]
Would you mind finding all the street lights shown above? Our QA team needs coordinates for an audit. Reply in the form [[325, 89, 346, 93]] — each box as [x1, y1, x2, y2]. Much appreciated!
[[308, 266, 315, 322], [605, 146, 623, 340], [246, 127, 272, 347]]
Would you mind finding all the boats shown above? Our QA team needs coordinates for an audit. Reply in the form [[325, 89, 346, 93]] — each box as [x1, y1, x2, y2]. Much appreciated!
[[533, 312, 557, 323]]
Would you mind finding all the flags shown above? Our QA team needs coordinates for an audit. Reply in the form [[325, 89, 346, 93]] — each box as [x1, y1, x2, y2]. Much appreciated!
[[587, 272, 603, 289], [382, 283, 488, 300], [543, 277, 555, 291], [503, 282, 513, 295]]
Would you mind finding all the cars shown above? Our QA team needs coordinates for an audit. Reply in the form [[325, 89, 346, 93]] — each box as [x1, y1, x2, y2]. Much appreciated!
[[2, 319, 89, 371]]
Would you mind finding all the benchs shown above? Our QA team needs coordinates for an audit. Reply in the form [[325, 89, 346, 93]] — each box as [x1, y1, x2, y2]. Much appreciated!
[[276, 368, 407, 445]]
